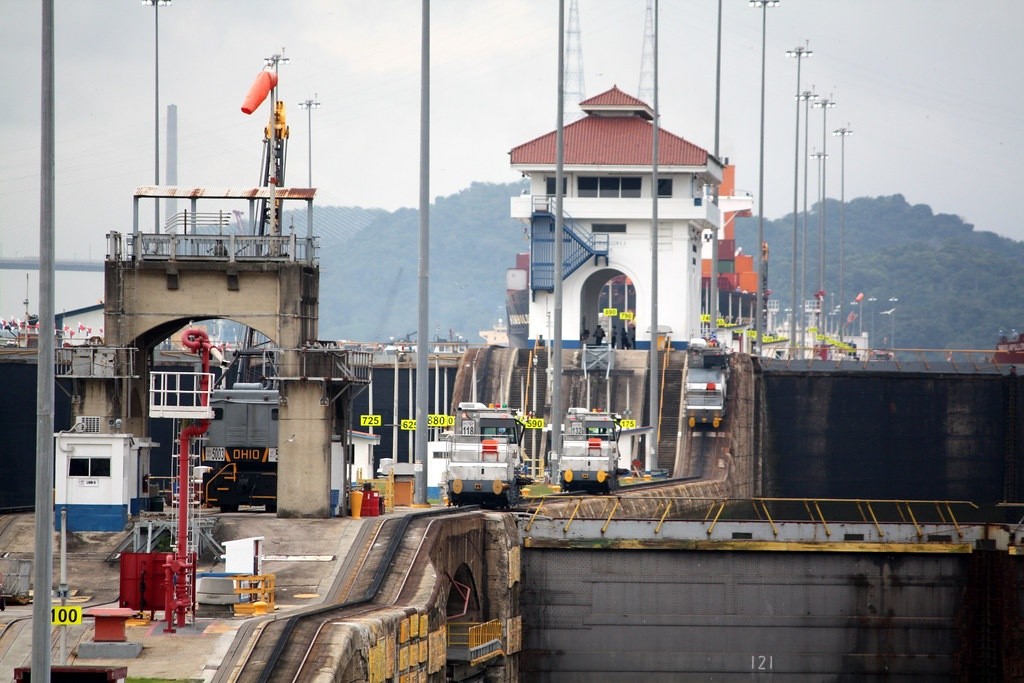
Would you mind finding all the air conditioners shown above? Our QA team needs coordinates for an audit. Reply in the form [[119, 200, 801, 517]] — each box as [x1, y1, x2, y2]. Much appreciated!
[[76, 416, 100, 433]]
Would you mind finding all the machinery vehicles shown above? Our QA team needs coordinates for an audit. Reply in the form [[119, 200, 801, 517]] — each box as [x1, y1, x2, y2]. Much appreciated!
[[561, 407, 624, 494], [685, 337, 730, 428], [444, 401, 525, 511]]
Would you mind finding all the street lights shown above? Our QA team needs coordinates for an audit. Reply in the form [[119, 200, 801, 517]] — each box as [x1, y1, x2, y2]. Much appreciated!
[[298, 93, 322, 267], [264, 46, 290, 256], [144, 1, 173, 252], [751, 0, 782, 355], [786, 38, 852, 357]]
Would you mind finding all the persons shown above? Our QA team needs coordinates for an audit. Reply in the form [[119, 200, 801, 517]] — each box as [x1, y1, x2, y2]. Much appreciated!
[[212, 240, 227, 256], [593, 325, 605, 346], [539, 335, 544, 346], [632, 327, 635, 350], [611, 325, 617, 348], [621, 328, 629, 349]]
